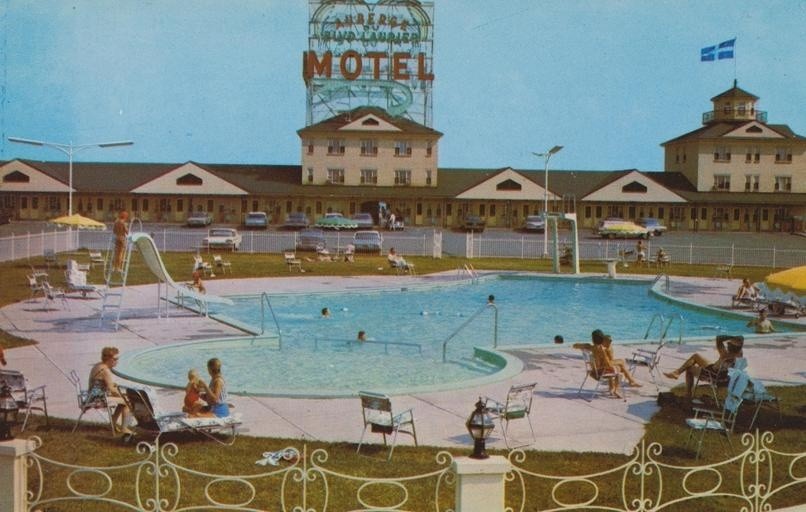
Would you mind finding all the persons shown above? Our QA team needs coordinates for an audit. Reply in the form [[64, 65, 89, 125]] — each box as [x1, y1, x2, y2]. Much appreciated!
[[695, 216, 700, 232], [384, 207, 391, 230], [746, 308, 783, 334], [603, 335, 617, 394], [488, 295, 495, 304], [637, 240, 648, 266], [321, 307, 329, 315], [573, 329, 643, 398], [215, 253, 227, 265], [318, 239, 330, 258], [184, 371, 202, 413], [189, 271, 206, 297], [657, 248, 667, 267], [389, 212, 396, 231], [343, 241, 354, 258], [737, 278, 758, 302], [182, 357, 234, 417], [750, 281, 760, 298], [86, 346, 136, 433], [554, 334, 564, 344], [192, 251, 209, 271], [389, 247, 411, 273], [358, 330, 365, 340], [113, 211, 131, 270], [663, 334, 747, 394]]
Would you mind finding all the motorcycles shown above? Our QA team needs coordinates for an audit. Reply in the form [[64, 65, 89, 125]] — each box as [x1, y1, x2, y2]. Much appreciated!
[[557, 239, 573, 267]]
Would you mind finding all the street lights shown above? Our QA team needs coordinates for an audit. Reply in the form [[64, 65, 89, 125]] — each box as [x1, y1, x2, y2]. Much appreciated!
[[531, 144, 563, 256], [7, 135, 132, 252]]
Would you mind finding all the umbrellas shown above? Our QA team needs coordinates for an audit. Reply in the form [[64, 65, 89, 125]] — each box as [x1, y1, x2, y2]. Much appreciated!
[[767, 264, 806, 293], [314, 215, 359, 260], [51, 211, 108, 269]]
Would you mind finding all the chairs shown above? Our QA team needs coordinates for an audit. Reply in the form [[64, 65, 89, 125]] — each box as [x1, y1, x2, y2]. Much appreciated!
[[193, 256, 216, 279], [285, 246, 357, 275], [112, 378, 248, 456], [64, 367, 125, 442], [683, 392, 748, 465], [622, 334, 672, 396], [652, 251, 673, 272], [213, 252, 235, 276], [468, 382, 542, 458], [691, 349, 748, 399], [579, 345, 634, 410], [728, 369, 784, 433], [12, 244, 108, 312], [0, 367, 58, 442], [388, 254, 414, 277], [344, 385, 424, 463], [710, 261, 737, 285], [730, 286, 806, 322]]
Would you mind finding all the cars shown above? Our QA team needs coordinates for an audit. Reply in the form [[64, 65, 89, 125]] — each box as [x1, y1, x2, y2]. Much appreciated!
[[245, 210, 269, 229], [283, 211, 382, 254], [595, 216, 667, 240], [185, 210, 215, 229], [523, 214, 544, 232], [460, 209, 486, 234], [203, 227, 243, 250], [0, 208, 10, 225]]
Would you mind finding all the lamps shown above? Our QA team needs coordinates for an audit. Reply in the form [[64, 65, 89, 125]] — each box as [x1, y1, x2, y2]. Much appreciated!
[[465, 399, 499, 462]]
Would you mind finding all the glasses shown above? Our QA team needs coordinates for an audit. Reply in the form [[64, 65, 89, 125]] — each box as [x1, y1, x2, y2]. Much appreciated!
[[111, 357, 118, 361]]
